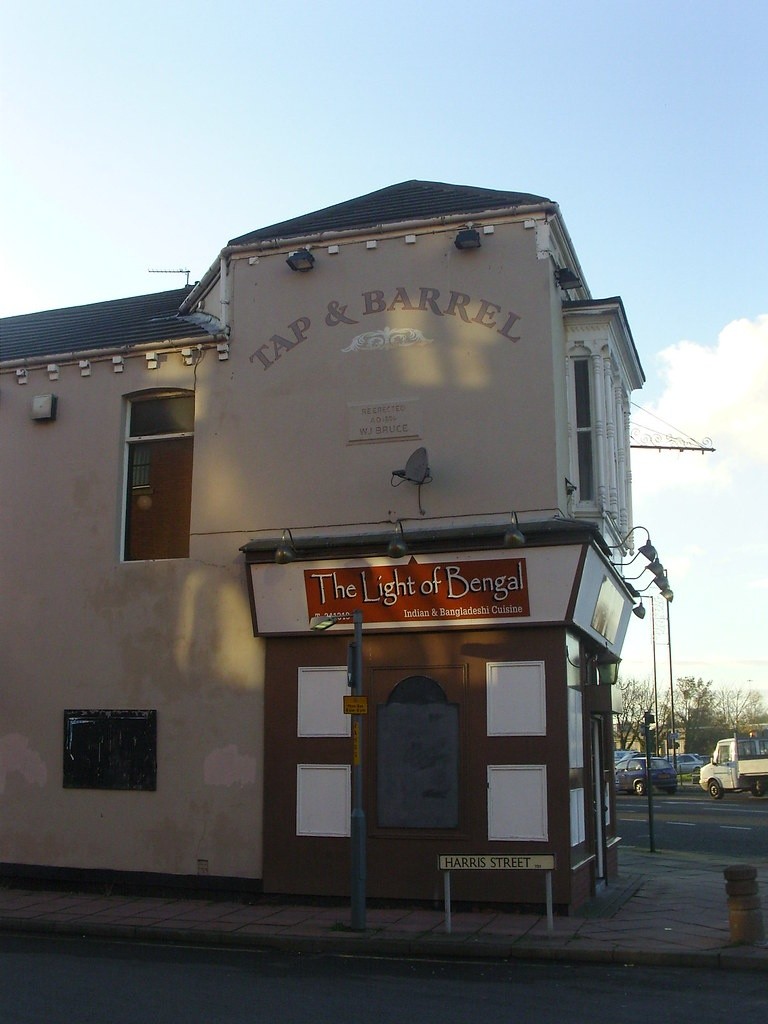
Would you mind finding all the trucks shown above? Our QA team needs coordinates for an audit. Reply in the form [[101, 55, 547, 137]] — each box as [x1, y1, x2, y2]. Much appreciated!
[[699, 729, 768, 800]]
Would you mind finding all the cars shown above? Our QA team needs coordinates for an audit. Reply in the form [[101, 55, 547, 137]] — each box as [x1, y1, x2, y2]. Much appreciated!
[[615, 751, 656, 766], [664, 754, 706, 774], [615, 756, 678, 796]]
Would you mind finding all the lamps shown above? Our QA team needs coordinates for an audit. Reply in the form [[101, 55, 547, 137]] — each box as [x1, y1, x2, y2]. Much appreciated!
[[634, 589, 674, 603], [635, 572, 670, 594], [606, 526, 658, 564], [30, 393, 57, 423], [286, 248, 314, 272], [621, 558, 663, 580], [554, 267, 584, 291], [566, 641, 622, 685], [632, 598, 646, 619], [386, 519, 407, 559], [454, 229, 481, 250], [504, 509, 525, 548], [274, 528, 295, 565]]
